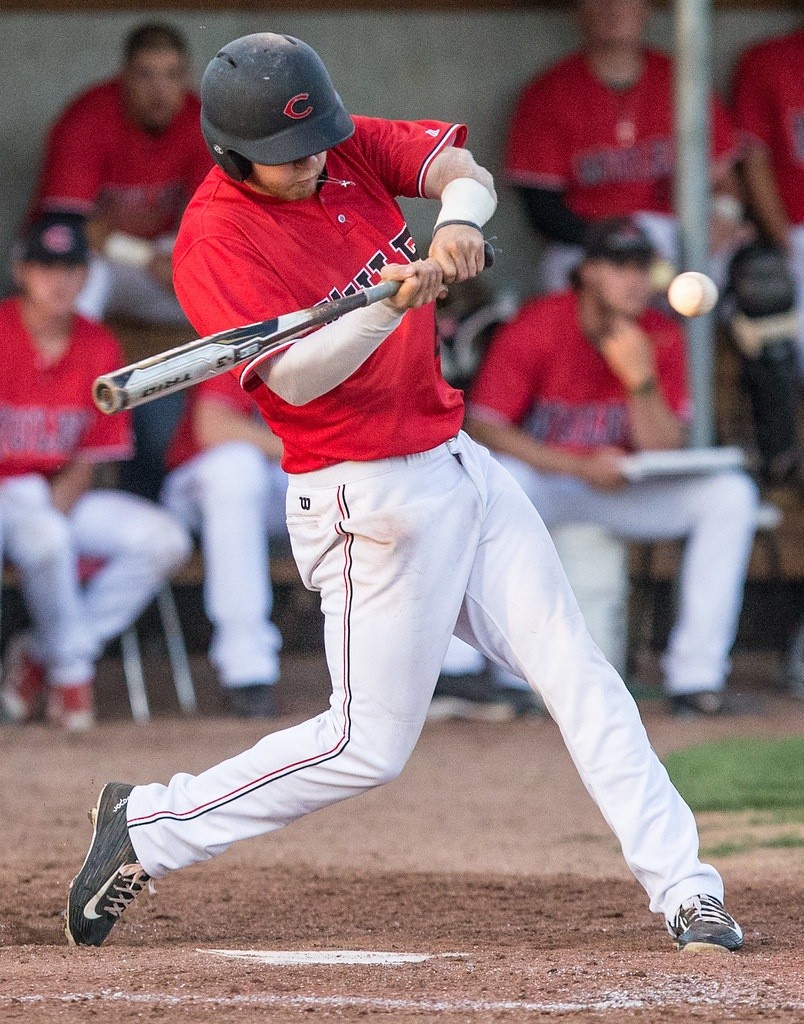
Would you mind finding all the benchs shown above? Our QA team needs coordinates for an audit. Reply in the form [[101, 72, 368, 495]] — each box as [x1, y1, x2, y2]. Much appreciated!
[[0, 312, 804, 668]]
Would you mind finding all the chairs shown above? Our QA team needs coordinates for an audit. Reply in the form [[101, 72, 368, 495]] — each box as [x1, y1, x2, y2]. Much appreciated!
[[71, 501, 791, 724]]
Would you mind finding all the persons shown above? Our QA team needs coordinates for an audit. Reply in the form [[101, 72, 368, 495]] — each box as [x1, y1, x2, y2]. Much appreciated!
[[159, 373, 520, 722], [0, 214, 192, 729], [64, 31, 744, 950], [40, 25, 215, 326], [462, 231, 758, 714], [499, 0, 744, 296], [734, 25, 804, 484]]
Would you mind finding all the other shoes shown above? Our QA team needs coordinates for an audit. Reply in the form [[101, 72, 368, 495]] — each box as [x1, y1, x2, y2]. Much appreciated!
[[232, 685, 283, 717], [2, 640, 46, 722], [670, 689, 731, 713], [424, 673, 513, 727], [43, 684, 97, 731]]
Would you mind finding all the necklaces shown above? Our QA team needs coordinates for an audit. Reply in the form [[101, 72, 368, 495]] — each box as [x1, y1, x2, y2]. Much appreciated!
[[317, 175, 350, 188]]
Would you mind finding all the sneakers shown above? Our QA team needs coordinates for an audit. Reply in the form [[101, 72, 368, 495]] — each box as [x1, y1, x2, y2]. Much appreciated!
[[65, 781, 156, 947], [670, 893, 743, 951]]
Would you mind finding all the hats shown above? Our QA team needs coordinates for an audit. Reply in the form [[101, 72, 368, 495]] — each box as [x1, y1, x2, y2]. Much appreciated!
[[583, 0, 643, 43], [16, 210, 87, 267], [584, 220, 654, 266]]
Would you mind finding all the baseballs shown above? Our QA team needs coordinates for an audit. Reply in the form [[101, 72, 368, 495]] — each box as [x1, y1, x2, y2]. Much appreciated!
[[667, 271, 719, 318]]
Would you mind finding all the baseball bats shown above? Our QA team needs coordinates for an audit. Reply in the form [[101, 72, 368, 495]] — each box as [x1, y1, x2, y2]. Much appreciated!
[[91, 241, 496, 416]]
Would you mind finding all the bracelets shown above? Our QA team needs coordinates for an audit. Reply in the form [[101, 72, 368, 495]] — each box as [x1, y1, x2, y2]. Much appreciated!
[[630, 375, 659, 396]]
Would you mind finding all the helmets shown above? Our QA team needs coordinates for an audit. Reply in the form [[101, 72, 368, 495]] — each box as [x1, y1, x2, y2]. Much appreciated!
[[199, 32, 354, 184]]
